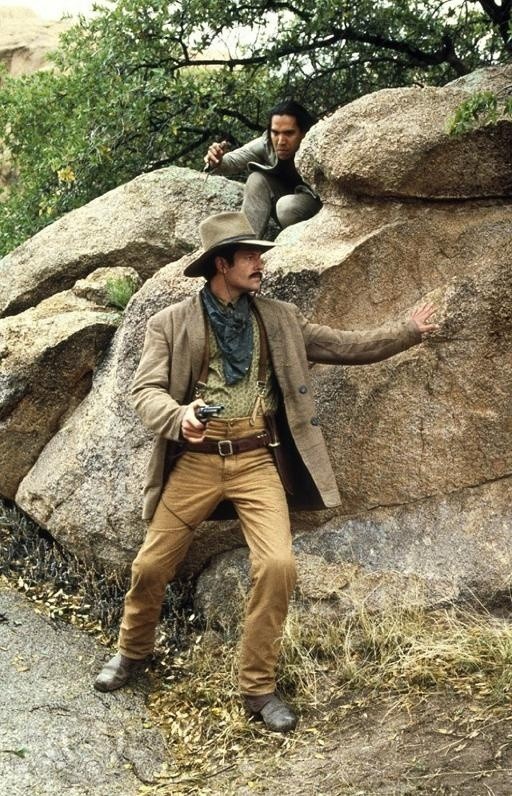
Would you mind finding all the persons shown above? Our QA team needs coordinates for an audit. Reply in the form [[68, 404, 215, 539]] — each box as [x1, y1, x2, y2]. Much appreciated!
[[202, 101, 322, 239], [95, 209, 442, 732]]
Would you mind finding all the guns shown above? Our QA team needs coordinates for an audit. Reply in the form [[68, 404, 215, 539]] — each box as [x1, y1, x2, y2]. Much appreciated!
[[195, 406, 223, 423]]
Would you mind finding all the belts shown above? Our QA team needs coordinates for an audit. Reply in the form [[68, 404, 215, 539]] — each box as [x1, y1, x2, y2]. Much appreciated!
[[183, 431, 271, 458]]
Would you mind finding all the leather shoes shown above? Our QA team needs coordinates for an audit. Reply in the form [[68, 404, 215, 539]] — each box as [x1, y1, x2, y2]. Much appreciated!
[[95, 651, 148, 692], [246, 694, 296, 733]]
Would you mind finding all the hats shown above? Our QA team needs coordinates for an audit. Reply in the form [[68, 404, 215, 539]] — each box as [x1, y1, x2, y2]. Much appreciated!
[[183, 210, 277, 277]]
